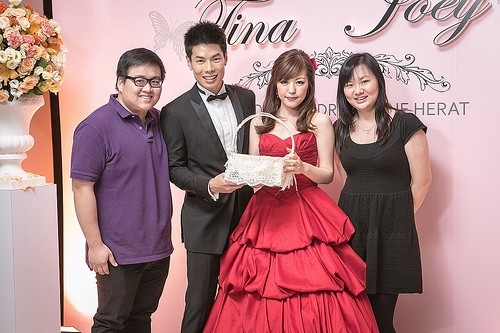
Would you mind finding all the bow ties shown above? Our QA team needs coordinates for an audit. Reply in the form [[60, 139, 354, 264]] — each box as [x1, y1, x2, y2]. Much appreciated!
[[207, 92, 228, 102]]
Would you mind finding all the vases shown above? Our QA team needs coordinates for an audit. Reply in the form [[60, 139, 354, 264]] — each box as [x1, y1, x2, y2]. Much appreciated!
[[0, 92, 45, 189]]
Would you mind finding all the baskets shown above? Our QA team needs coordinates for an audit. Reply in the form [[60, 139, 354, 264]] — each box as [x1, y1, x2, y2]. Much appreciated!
[[224, 112, 299, 193]]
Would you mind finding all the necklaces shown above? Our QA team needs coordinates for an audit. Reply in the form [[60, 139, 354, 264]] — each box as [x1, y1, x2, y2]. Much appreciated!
[[352, 115, 377, 134]]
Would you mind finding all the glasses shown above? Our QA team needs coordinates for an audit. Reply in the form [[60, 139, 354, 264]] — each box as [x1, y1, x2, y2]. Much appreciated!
[[117, 74, 164, 88]]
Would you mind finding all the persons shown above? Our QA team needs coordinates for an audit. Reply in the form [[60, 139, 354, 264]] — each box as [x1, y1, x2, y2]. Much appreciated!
[[70, 48, 173, 333], [332, 53, 432, 333], [204, 48, 379, 333], [160, 21, 256, 333]]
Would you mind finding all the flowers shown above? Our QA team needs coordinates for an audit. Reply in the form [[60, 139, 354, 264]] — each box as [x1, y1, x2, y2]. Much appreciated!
[[0, 0, 68, 103]]
